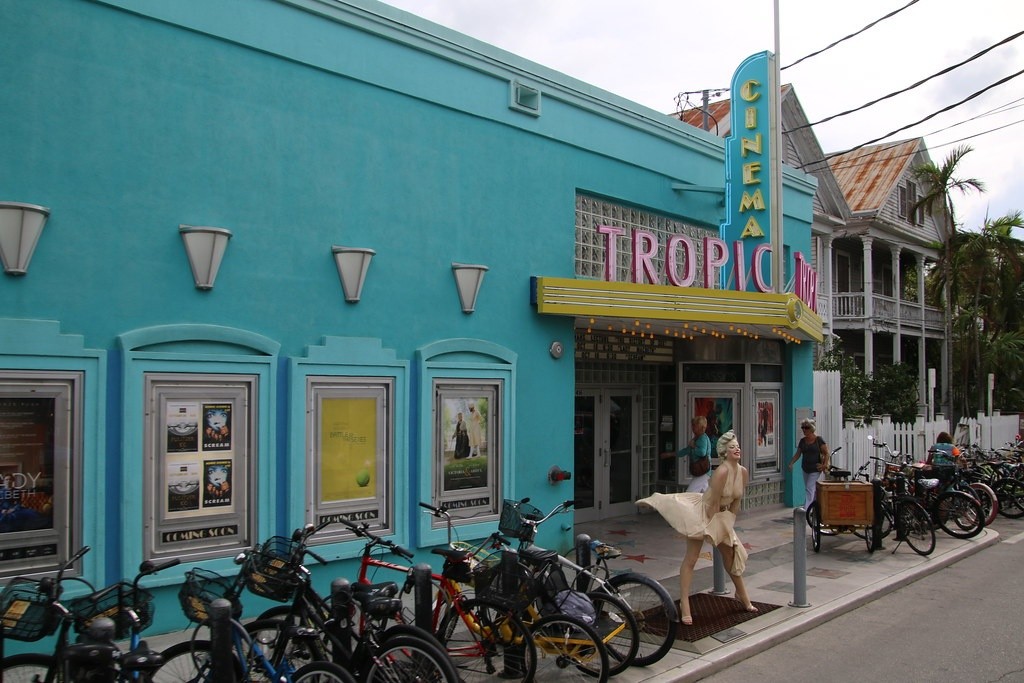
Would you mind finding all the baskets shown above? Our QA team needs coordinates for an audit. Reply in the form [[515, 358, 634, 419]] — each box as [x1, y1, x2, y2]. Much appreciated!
[[67, 581, 154, 640], [0, 577, 63, 643], [914, 470, 944, 492], [472, 550, 550, 614], [257, 536, 300, 580], [178, 567, 242, 626], [245, 551, 298, 603], [449, 541, 501, 587], [498, 499, 544, 540], [933, 465, 955, 483]]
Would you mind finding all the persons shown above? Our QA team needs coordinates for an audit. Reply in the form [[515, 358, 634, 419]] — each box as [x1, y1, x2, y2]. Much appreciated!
[[788, 418, 830, 511], [761, 401, 770, 447], [926, 432, 968, 491], [660, 416, 711, 494], [633, 430, 757, 628], [452, 403, 483, 460]]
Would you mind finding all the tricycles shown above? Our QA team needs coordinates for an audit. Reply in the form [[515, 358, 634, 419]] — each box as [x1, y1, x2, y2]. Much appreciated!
[[804, 446, 877, 555]]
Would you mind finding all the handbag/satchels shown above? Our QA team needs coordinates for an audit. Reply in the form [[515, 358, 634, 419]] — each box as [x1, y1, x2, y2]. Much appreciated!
[[689, 434, 711, 477], [816, 437, 831, 480]]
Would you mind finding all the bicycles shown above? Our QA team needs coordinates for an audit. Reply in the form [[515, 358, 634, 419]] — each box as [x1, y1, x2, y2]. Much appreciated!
[[852, 434, 1024, 557], [1, 494, 681, 683]]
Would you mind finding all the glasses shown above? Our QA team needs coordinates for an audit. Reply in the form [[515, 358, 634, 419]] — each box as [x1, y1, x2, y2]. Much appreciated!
[[801, 426, 810, 430]]
